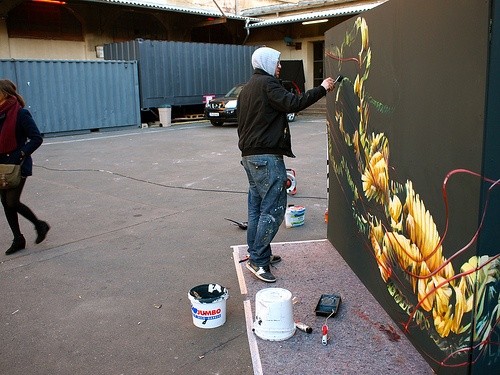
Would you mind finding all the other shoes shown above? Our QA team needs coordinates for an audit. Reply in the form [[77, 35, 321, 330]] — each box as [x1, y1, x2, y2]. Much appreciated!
[[5, 234, 26, 255], [35, 221, 49, 244]]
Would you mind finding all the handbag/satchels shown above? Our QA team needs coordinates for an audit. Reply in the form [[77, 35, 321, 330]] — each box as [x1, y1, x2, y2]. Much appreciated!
[[0, 164, 22, 189]]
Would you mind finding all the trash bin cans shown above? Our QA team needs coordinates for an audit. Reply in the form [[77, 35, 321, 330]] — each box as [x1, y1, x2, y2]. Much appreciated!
[[158, 104, 171, 128]]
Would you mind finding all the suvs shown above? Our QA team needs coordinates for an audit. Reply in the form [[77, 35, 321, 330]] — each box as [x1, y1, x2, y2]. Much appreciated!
[[203, 79, 301, 127]]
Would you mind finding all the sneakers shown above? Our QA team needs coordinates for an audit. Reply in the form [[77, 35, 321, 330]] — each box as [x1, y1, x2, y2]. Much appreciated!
[[247, 254, 282, 263], [246, 259, 277, 283]]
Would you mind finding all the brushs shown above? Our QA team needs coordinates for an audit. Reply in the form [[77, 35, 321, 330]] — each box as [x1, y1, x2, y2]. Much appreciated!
[[319, 304, 336, 346], [334, 75, 343, 84]]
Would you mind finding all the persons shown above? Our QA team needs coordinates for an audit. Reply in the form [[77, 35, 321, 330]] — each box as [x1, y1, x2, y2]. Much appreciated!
[[237, 46, 335, 282], [0, 78, 51, 255]]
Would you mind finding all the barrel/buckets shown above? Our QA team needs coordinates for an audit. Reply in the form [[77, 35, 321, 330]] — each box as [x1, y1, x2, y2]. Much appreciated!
[[253, 287, 296, 341], [187, 284, 229, 329], [285, 205, 307, 228]]
